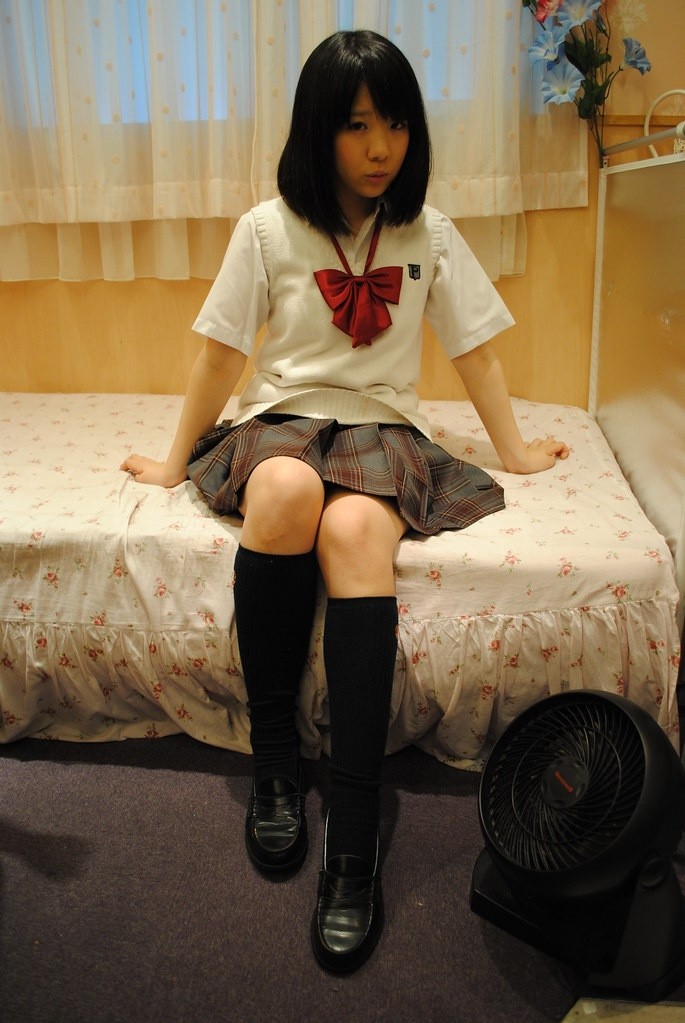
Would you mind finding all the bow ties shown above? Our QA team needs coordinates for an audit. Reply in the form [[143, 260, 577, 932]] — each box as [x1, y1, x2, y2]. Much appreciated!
[[313, 208, 404, 348]]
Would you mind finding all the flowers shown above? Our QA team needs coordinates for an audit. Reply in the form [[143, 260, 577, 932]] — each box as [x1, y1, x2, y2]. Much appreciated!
[[522, 0, 651, 168]]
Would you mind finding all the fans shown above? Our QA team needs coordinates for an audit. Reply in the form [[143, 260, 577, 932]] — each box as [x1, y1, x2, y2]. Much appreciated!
[[469, 692, 685, 1003]]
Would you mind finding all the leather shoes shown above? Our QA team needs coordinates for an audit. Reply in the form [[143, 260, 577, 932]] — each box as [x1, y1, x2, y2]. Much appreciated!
[[244, 763, 309, 881], [311, 804, 383, 976]]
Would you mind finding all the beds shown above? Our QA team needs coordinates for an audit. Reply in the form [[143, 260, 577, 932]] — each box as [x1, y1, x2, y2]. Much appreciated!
[[0, 389, 675, 778]]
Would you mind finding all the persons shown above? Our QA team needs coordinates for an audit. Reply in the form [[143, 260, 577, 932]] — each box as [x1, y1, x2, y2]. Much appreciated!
[[122, 30, 569, 969]]
